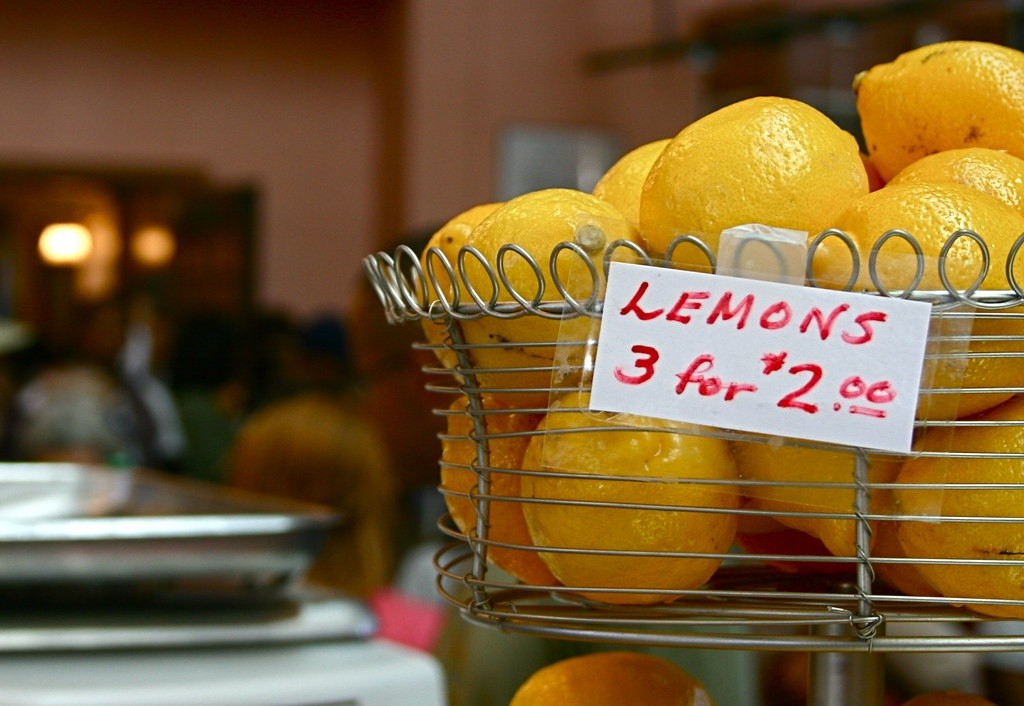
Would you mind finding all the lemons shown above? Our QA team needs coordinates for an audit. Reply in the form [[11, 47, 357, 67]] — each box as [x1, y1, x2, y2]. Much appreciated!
[[503, 647, 710, 706], [409, 40, 1024, 622]]
